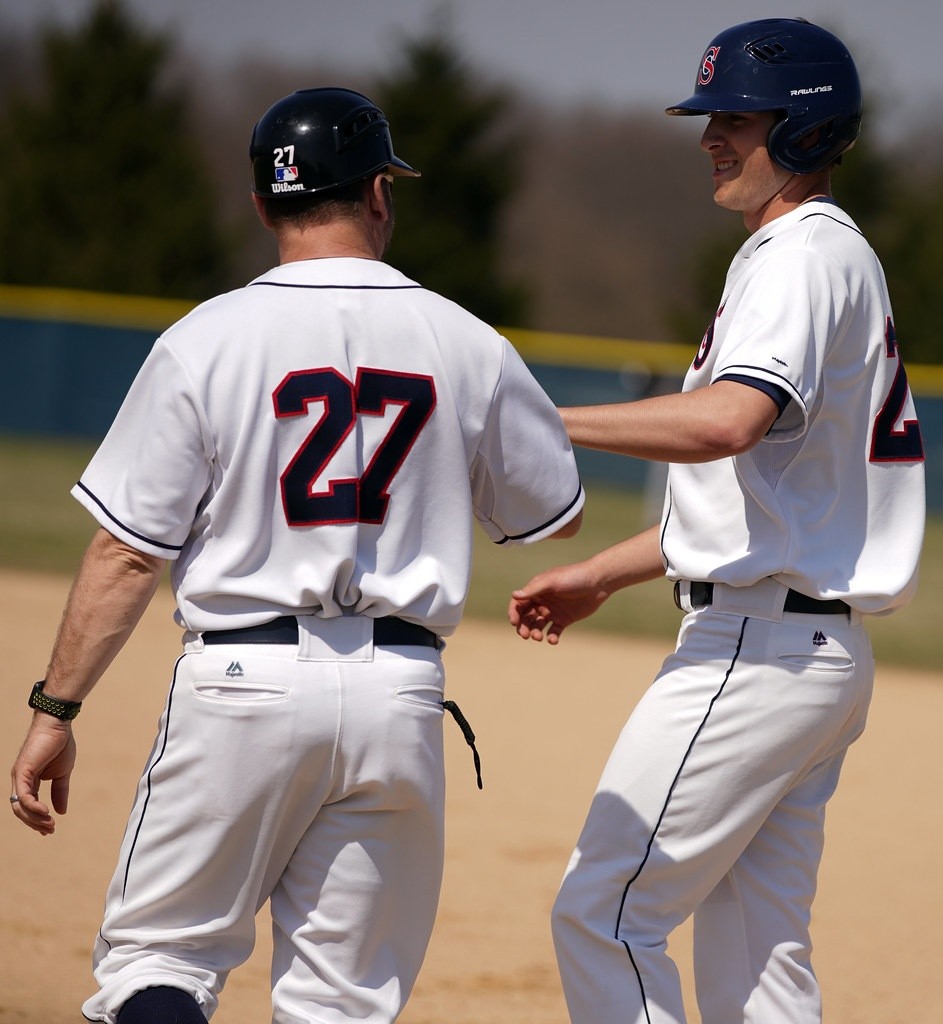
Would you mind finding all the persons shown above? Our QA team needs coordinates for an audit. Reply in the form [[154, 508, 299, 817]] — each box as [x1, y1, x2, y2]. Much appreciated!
[[10, 86, 586, 1024], [505, 13, 928, 1021]]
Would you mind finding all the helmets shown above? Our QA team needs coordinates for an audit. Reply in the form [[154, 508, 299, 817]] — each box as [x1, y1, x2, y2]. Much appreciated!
[[664, 18, 861, 177], [251, 88, 420, 198]]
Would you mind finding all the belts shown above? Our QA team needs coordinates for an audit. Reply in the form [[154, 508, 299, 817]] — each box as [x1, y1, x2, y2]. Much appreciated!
[[675, 579, 850, 617], [205, 614, 438, 650]]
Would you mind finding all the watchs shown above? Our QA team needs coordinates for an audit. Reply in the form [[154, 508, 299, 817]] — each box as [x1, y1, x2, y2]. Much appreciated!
[[27, 679, 82, 721]]
